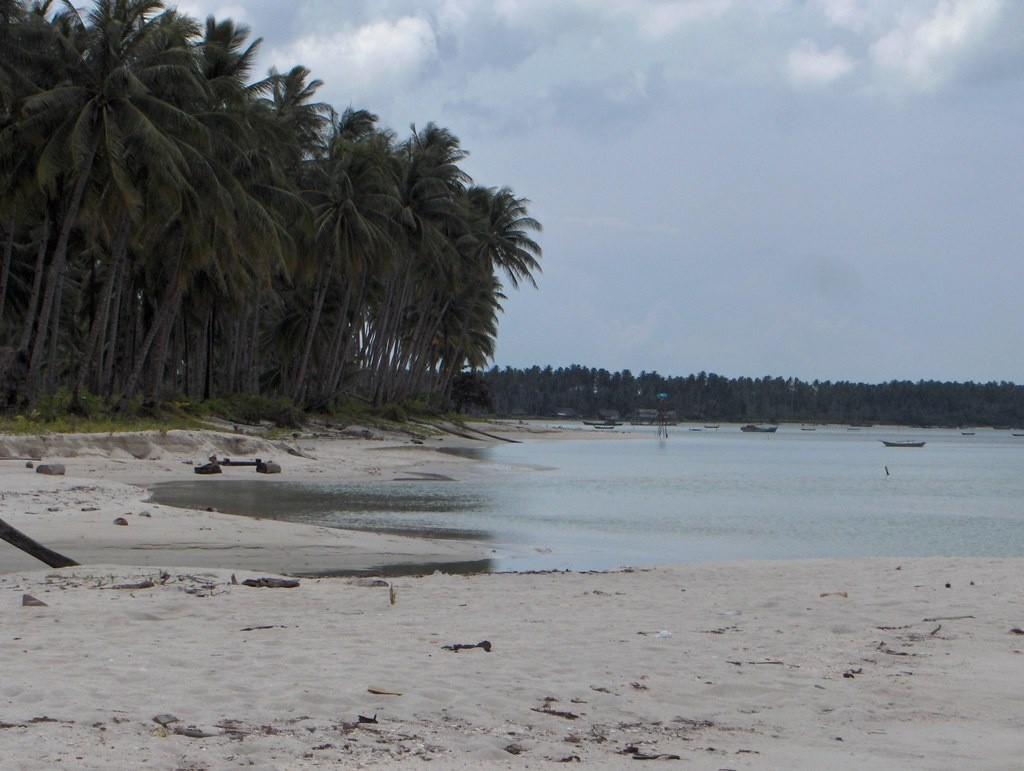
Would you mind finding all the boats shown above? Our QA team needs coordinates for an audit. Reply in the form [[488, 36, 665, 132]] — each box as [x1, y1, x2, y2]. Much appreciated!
[[880, 439, 926, 448], [689, 427, 703, 432], [740, 422, 779, 432], [582, 419, 623, 428], [961, 432, 975, 436], [1012, 433, 1024, 436], [704, 423, 720, 428], [593, 425, 616, 430]]
